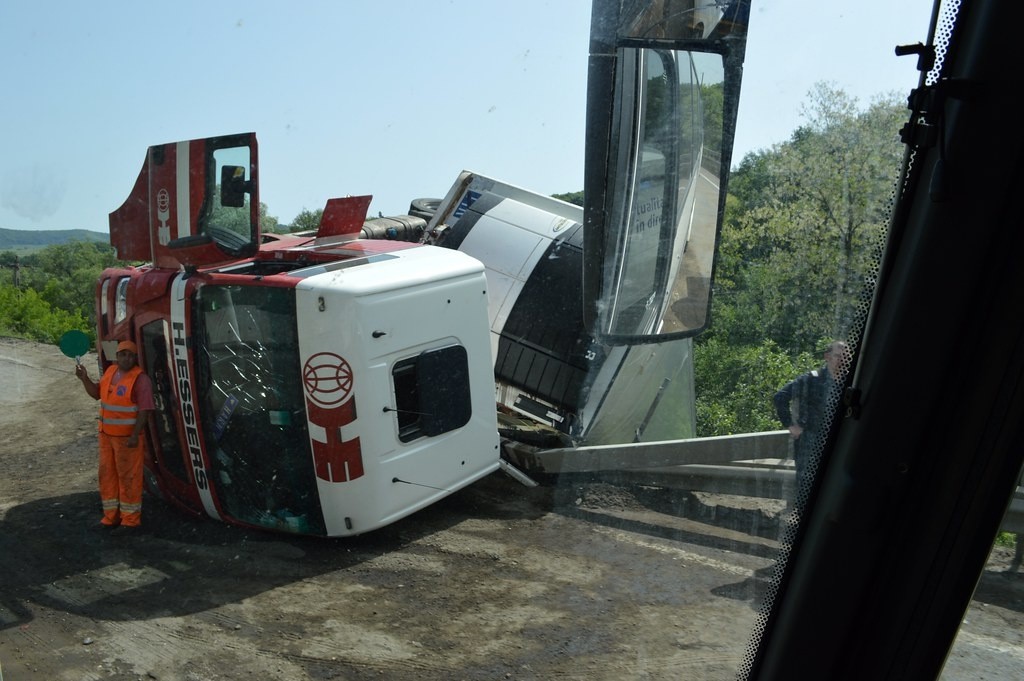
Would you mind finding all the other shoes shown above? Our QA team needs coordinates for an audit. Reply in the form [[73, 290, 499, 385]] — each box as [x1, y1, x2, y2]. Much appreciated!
[[110, 524, 142, 535], [91, 523, 116, 531]]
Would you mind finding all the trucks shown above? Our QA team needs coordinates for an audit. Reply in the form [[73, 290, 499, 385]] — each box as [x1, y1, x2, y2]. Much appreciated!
[[91, 131, 596, 544]]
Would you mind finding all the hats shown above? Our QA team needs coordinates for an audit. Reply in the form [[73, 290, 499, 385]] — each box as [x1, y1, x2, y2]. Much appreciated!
[[116, 340, 137, 355]]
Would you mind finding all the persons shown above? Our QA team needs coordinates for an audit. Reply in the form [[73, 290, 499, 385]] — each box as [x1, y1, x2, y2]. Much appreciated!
[[774, 341, 853, 519], [76, 341, 156, 536]]
